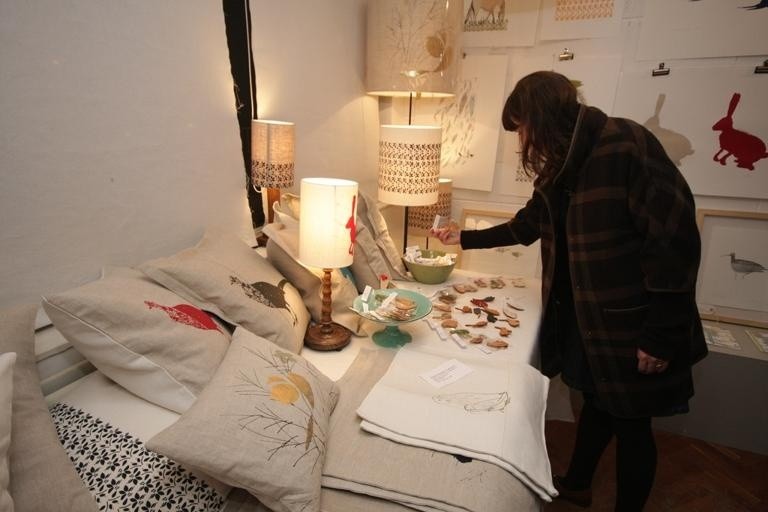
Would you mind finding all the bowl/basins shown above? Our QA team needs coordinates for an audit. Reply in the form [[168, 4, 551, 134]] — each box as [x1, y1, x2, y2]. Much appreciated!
[[400, 249, 456, 285]]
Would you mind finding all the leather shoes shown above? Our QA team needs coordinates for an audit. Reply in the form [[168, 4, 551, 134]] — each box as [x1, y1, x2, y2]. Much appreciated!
[[551, 474, 594, 509]]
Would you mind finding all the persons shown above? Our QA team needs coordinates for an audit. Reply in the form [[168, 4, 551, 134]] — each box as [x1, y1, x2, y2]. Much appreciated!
[[428, 71, 709, 512]]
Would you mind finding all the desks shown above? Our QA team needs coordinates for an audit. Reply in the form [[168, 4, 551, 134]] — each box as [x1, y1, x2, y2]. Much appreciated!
[[0, 261, 542, 512]]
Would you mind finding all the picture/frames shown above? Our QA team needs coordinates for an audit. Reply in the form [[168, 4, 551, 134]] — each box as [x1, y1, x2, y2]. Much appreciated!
[[695, 209, 768, 329], [456, 209, 542, 279]]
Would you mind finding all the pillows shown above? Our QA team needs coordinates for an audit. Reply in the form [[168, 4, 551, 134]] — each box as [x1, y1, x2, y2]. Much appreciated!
[[262, 200, 372, 338], [359, 192, 411, 284], [141, 226, 312, 356], [283, 193, 393, 289], [2, 303, 100, 512], [40, 265, 232, 413], [145, 325, 340, 512]]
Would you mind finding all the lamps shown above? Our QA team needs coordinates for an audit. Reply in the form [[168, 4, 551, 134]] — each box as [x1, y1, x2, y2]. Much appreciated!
[[378, 125, 443, 253], [299, 177, 358, 352], [251, 119, 296, 247], [407, 179, 452, 251], [364, 1, 464, 127]]
